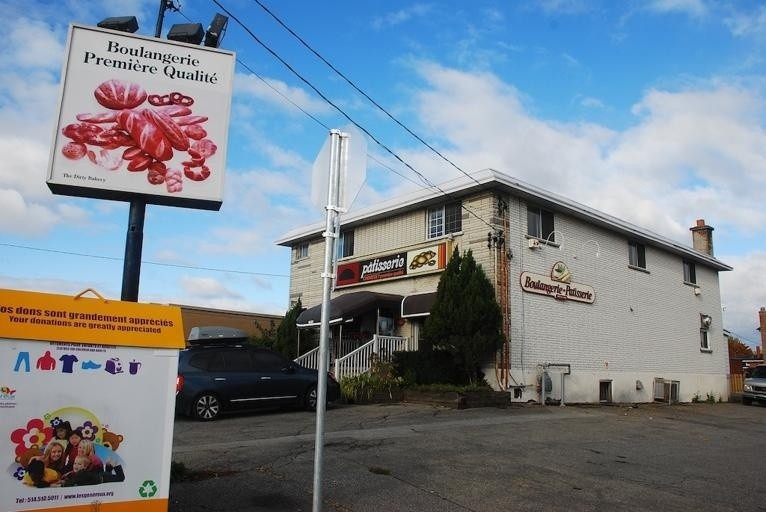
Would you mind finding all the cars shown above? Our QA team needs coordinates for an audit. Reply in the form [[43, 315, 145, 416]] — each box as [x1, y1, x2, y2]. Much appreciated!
[[175, 341, 340, 423], [741, 364, 766, 403]]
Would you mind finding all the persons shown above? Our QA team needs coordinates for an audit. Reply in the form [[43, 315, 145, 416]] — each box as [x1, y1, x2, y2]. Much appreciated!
[[21, 421, 125, 488]]
